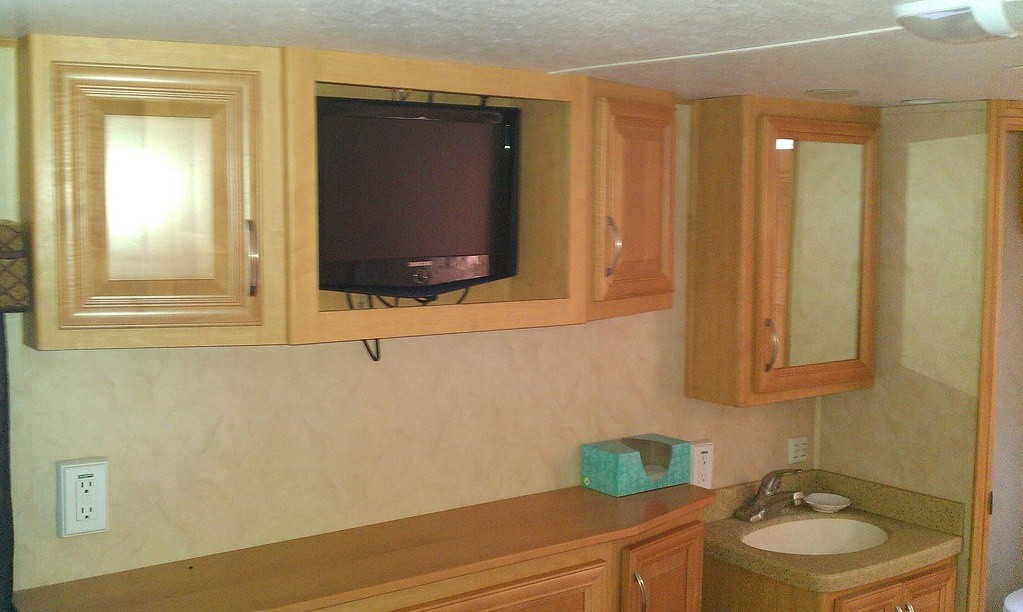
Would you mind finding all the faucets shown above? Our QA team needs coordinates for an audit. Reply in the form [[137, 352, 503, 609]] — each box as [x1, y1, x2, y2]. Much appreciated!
[[751, 469, 806, 512]]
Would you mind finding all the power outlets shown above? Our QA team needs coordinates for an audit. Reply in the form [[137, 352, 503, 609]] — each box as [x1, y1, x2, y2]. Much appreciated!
[[690, 440, 714, 491], [56, 456, 109, 539]]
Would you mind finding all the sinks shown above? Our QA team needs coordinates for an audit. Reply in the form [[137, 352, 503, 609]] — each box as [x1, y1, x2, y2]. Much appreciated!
[[741, 515, 890, 556]]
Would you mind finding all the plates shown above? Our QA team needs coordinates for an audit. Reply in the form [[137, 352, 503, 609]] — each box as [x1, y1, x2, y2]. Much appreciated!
[[804, 493, 852, 513]]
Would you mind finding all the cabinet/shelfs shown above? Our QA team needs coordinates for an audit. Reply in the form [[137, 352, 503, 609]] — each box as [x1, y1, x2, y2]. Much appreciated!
[[701, 554, 960, 612], [16, 32, 685, 352], [12, 485, 716, 611], [684, 94, 882, 410]]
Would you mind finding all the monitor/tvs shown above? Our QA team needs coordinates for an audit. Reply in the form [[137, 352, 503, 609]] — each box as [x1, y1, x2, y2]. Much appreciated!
[[318, 95, 521, 300]]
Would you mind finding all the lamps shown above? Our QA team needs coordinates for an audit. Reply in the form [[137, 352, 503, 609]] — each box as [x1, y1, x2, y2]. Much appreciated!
[[892, 1, 1021, 45]]
